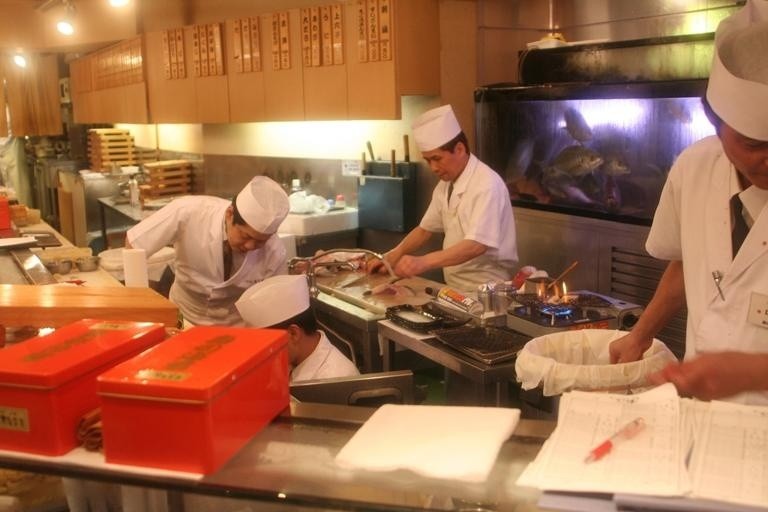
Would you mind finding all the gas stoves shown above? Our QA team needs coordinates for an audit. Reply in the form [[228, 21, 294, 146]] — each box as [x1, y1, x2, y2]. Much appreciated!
[[506, 289, 646, 339]]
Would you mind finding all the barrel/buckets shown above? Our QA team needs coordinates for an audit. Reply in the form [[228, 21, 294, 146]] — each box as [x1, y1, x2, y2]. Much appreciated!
[[98, 248, 126, 281]]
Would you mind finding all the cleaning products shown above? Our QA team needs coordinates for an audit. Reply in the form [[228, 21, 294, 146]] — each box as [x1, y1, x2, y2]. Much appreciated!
[[128, 176, 139, 205]]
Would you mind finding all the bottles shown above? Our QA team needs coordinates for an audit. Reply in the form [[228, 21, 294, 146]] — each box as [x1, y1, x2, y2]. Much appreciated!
[[335, 195, 345, 207], [291, 179, 301, 194], [129, 174, 139, 206], [425, 286, 484, 317]]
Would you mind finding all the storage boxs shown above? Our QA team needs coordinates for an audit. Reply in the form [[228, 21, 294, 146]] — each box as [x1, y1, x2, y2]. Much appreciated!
[[96, 325, 291, 474], [0, 316, 167, 456]]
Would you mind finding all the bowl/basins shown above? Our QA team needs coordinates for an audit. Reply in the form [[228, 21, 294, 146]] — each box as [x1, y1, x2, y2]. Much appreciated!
[[120, 166, 138, 174], [45, 261, 72, 274], [75, 256, 101, 271]]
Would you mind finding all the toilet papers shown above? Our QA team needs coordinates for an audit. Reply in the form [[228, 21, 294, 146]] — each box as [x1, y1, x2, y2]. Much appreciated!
[[122, 246, 150, 288]]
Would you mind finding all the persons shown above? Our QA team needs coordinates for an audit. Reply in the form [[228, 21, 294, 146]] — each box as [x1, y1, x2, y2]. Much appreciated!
[[124, 174, 290, 329], [364, 104, 519, 293], [233, 273, 362, 382], [607, 0, 767, 409]]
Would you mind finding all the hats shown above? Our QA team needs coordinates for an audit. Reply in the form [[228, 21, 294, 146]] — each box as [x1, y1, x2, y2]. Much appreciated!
[[411, 104, 463, 153], [703, 0, 768, 143], [234, 274, 309, 331], [235, 175, 291, 235]]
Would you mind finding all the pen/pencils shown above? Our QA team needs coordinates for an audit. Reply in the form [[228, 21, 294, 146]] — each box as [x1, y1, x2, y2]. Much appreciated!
[[583, 417, 646, 466]]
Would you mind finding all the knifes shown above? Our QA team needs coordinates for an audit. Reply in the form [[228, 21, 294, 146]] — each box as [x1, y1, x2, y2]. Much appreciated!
[[336, 269, 377, 289], [363, 275, 404, 296]]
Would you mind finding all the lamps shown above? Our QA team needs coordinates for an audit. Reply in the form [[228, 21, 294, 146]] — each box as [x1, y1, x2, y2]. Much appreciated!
[[58, 0, 74, 34]]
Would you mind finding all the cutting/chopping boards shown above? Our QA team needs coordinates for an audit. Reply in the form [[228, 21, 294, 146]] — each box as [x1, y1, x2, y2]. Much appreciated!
[[302, 263, 448, 316]]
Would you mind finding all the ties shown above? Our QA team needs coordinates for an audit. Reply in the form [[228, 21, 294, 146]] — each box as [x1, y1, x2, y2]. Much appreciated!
[[447, 183, 453, 206], [222, 241, 233, 282], [732, 195, 750, 261]]
